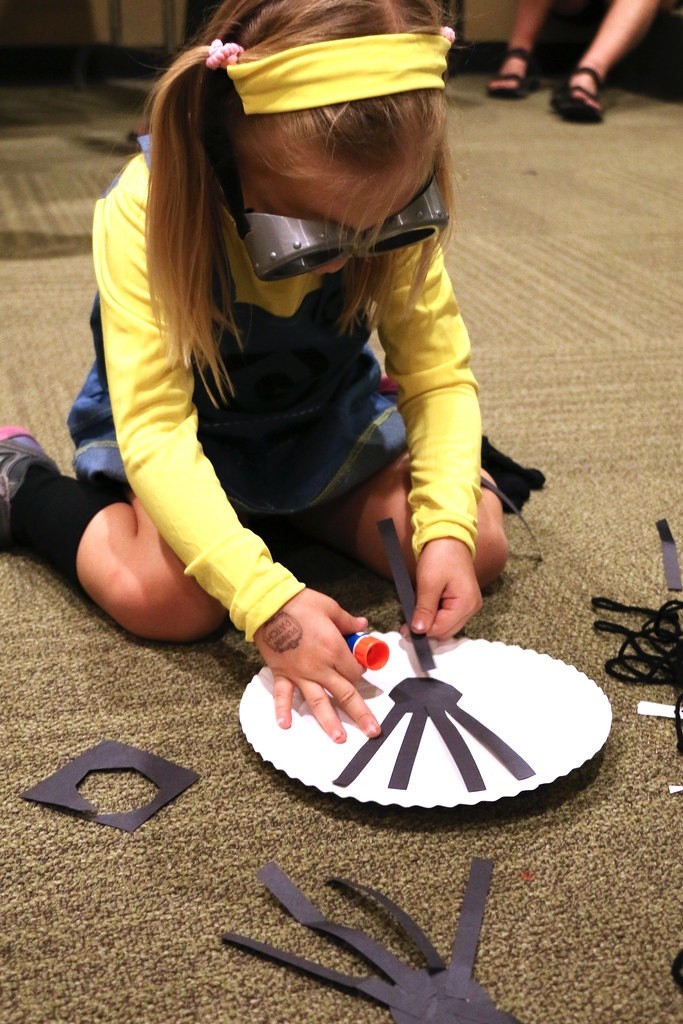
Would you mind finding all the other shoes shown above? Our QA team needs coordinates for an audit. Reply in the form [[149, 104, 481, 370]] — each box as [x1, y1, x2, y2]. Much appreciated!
[[0, 424, 60, 544]]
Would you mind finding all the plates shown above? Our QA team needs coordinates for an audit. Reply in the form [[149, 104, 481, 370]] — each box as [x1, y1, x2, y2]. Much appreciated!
[[238, 629, 613, 809]]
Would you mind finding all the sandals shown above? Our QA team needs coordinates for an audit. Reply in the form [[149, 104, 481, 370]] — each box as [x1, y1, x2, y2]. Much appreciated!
[[555, 67, 605, 122], [488, 47, 540, 98]]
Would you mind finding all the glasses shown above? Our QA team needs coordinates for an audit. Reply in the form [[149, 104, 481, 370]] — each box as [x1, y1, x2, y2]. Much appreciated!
[[218, 165, 452, 283]]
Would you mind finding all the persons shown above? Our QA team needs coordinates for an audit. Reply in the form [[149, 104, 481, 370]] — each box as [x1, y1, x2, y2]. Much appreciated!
[[0, 0, 547, 744], [486, 0, 664, 124]]
